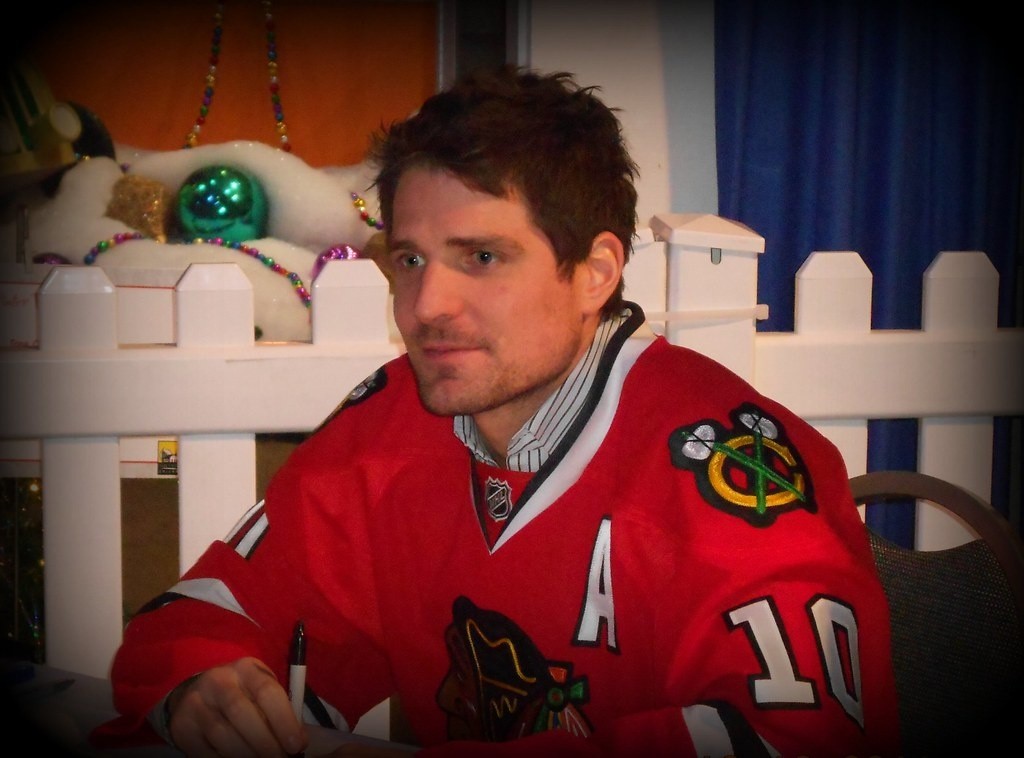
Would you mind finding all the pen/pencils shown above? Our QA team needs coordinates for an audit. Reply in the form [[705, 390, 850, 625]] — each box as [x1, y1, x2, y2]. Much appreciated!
[[284, 619, 307, 716]]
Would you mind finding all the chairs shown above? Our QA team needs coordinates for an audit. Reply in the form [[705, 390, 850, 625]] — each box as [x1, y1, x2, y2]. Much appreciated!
[[849, 471, 1024, 758]]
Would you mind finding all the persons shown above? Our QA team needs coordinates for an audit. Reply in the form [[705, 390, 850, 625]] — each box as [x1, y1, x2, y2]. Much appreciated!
[[110, 66, 900, 758]]
